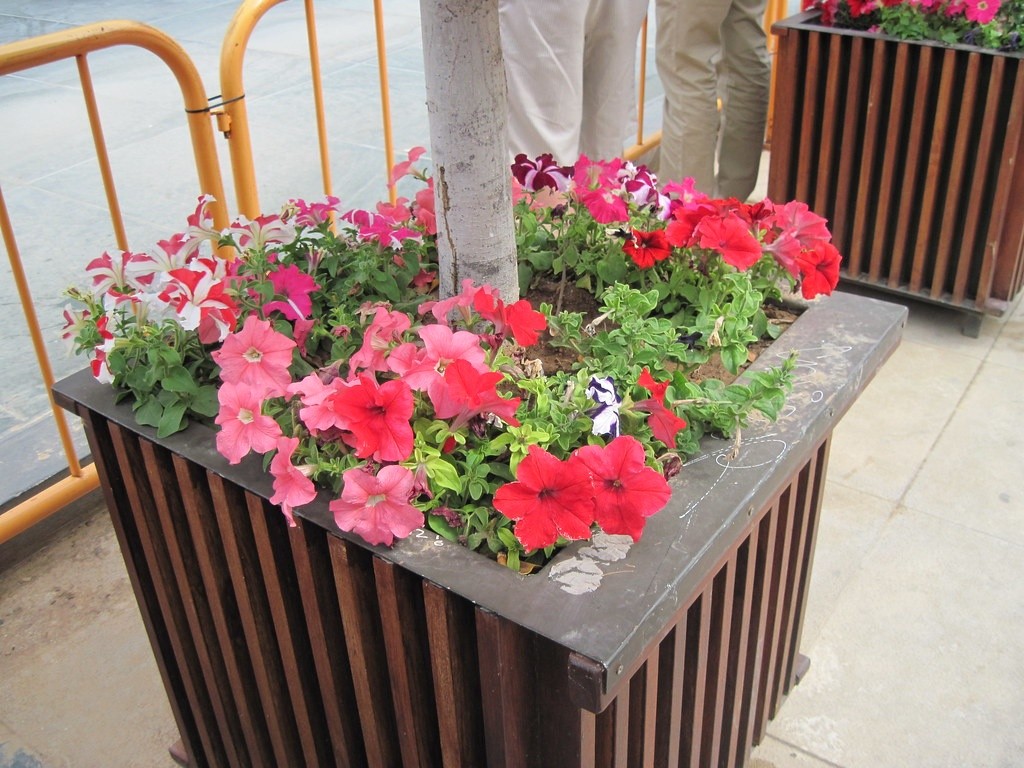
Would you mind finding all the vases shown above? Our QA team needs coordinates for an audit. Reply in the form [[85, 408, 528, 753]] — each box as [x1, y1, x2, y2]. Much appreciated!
[[48, 284, 912, 768], [764, 19, 1024, 341]]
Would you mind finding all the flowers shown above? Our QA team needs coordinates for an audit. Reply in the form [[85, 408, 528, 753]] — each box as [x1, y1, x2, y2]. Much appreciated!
[[62, 141, 844, 545], [804, 0, 1024, 55]]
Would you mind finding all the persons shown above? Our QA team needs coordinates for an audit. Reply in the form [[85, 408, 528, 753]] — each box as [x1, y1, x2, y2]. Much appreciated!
[[499, 0, 650, 167], [656, 0, 772, 203]]
